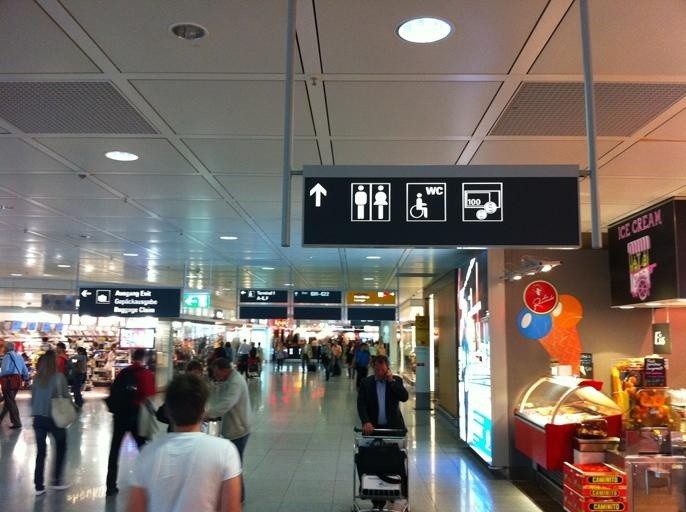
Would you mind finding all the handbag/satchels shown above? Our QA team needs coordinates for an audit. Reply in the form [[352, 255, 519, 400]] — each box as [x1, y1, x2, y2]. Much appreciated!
[[50, 398, 76, 429], [0, 373, 21, 391], [356, 438, 407, 483]]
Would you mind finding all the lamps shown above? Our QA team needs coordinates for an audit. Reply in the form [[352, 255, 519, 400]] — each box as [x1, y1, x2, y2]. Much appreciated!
[[496, 250, 560, 286]]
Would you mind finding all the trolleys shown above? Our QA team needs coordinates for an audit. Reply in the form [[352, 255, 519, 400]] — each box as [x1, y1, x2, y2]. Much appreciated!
[[626, 235, 657, 302], [246, 355, 263, 380], [351, 425, 411, 512]]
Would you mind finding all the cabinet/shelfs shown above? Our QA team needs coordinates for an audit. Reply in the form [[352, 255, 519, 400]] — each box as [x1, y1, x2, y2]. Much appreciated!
[[2, 328, 119, 387], [510, 373, 628, 475]]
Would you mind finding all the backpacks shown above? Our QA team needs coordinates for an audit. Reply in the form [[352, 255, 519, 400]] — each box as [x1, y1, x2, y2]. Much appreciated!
[[105, 367, 146, 416]]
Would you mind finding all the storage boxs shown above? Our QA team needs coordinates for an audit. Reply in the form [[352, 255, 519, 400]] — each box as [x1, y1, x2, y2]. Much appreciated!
[[558, 437, 631, 512]]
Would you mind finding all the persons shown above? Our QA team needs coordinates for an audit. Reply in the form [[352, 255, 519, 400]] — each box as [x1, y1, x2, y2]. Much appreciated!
[[415, 192, 429, 219], [127, 374, 244, 512], [354, 183, 369, 218], [31, 347, 75, 497], [357, 354, 411, 511], [106, 346, 159, 498], [204, 356, 252, 505], [2, 328, 394, 441], [374, 184, 388, 218]]
